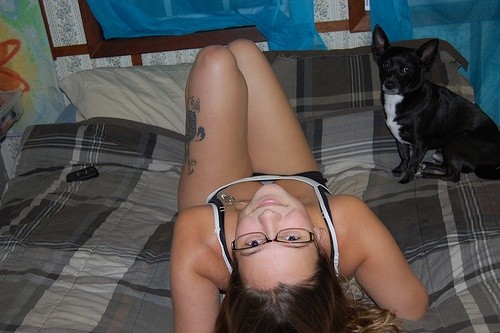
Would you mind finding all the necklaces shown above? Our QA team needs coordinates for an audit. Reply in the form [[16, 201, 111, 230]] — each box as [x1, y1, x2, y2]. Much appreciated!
[[221, 195, 247, 215]]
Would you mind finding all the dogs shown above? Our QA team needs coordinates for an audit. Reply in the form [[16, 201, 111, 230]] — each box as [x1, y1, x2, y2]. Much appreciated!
[[371, 23, 499, 185]]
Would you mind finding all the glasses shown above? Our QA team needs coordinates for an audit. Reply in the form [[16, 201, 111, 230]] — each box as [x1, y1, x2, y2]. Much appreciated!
[[230, 228, 319, 263]]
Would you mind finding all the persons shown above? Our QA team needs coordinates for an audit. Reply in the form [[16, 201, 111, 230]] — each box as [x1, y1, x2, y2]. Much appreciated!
[[170, 38, 430, 333]]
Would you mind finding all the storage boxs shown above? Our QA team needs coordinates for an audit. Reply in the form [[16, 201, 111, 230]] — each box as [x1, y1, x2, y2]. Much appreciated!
[[0, 89, 24, 142]]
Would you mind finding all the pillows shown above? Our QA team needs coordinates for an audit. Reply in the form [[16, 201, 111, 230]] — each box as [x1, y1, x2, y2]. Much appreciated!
[[59, 62, 193, 137]]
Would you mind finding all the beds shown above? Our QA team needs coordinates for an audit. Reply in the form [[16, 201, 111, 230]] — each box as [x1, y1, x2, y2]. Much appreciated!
[[0, 37, 500, 333]]
